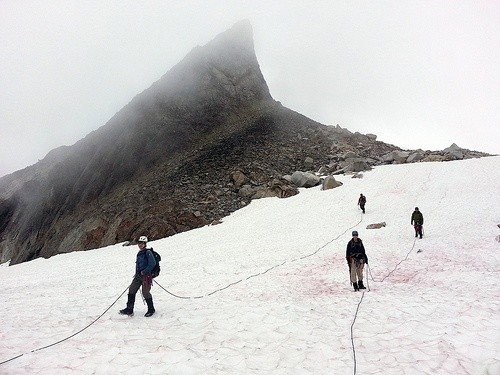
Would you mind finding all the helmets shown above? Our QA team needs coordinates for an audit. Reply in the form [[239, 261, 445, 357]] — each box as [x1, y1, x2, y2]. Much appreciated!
[[138, 236, 147, 242]]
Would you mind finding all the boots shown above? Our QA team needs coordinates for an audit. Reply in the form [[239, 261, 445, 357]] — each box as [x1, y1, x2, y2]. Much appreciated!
[[121, 294, 135, 314], [353, 282, 358, 289], [145, 297, 155, 317], [358, 281, 366, 289]]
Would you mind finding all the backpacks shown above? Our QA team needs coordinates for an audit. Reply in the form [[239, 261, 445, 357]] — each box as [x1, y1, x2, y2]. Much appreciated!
[[145, 248, 161, 278], [363, 196, 366, 203]]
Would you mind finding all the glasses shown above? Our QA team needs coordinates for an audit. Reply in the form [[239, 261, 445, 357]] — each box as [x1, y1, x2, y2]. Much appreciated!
[[353, 234, 358, 236]]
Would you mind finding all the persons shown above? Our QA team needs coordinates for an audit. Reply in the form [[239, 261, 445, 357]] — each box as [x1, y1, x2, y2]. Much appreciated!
[[119, 235, 155, 317], [411, 207, 423, 239], [358, 193, 366, 214], [346, 230, 368, 292]]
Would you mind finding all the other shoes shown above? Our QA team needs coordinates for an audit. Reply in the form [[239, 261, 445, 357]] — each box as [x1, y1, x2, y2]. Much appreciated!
[[415, 234, 418, 237]]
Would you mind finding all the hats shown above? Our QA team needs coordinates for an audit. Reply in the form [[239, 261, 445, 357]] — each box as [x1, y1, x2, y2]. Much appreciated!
[[352, 231, 357, 234], [360, 194, 363, 195], [415, 207, 418, 210]]
